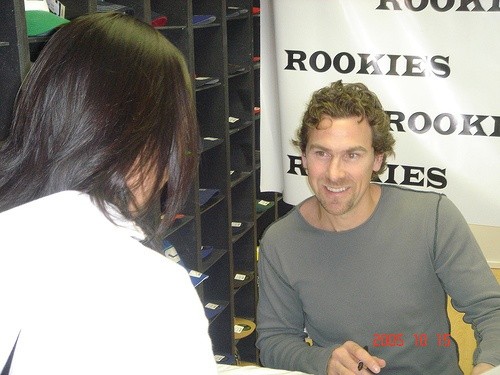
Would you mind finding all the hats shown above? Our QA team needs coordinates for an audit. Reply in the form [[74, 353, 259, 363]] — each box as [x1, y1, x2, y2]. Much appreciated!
[[193, 14, 216, 26], [150, 10, 168, 27], [24, 0, 72, 38], [97, 0, 136, 18], [226, 6, 249, 19], [141, 58, 277, 368]]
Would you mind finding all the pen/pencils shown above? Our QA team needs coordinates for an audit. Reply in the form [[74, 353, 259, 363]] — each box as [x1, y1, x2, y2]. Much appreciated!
[[358, 344, 369, 370]]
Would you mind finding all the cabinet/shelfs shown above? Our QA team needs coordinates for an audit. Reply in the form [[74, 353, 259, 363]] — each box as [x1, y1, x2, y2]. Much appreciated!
[[0, 0, 294, 366]]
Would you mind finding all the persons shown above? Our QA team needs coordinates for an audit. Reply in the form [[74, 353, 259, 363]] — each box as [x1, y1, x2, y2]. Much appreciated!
[[255, 79, 500, 375], [0, 15, 216, 375]]
